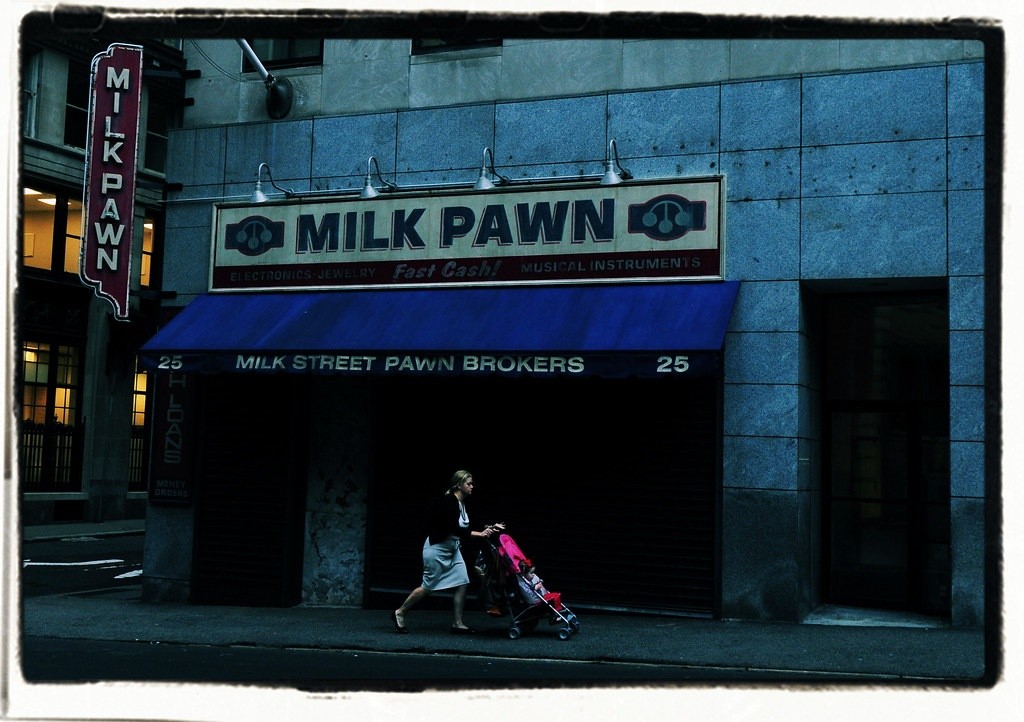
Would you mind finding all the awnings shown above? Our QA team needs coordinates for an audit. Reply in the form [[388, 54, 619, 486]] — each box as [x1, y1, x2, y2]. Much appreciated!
[[144, 282, 742, 353]]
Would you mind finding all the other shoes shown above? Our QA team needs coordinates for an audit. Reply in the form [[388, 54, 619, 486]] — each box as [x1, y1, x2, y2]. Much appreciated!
[[396, 610, 405, 627], [452, 624, 469, 629]]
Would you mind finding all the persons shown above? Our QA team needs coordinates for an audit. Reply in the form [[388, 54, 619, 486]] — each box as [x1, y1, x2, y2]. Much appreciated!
[[395, 470, 494, 630], [517, 559, 576, 624]]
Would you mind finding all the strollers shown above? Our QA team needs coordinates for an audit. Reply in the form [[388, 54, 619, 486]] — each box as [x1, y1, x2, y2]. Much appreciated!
[[485, 522, 581, 642]]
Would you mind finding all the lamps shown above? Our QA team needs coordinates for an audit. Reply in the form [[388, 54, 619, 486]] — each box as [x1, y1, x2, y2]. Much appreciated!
[[472, 147, 510, 190], [359, 157, 398, 199], [249, 163, 294, 204], [599, 140, 630, 185]]
